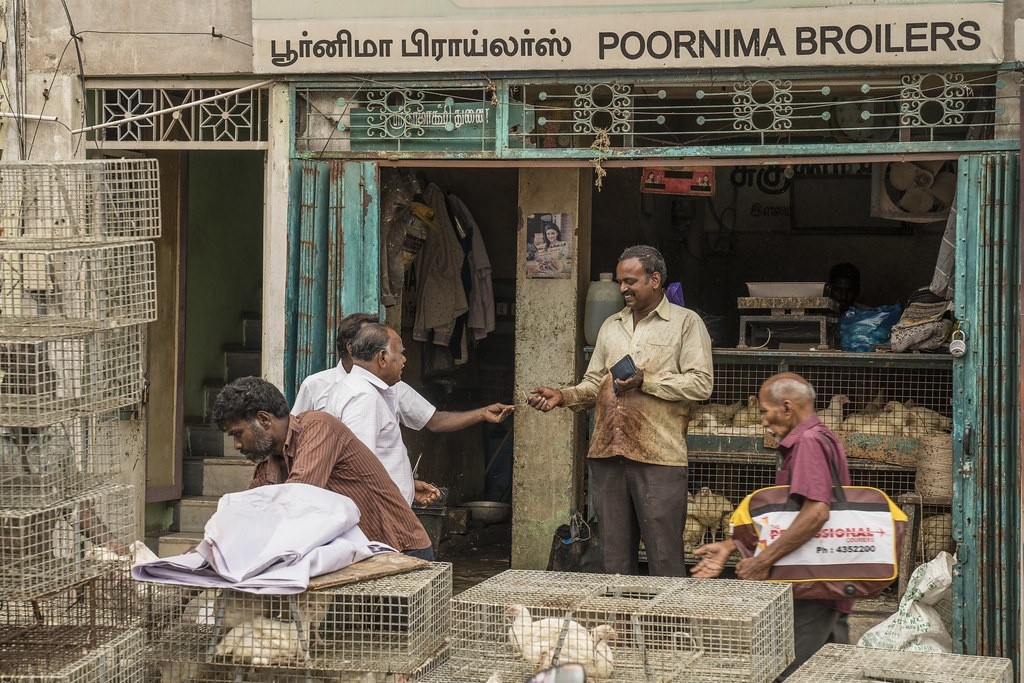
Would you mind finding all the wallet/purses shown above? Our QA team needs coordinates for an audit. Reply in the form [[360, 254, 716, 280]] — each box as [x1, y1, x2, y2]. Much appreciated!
[[610, 356, 636, 397]]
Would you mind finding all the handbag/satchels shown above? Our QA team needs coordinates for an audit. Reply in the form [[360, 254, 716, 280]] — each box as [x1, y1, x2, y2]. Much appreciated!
[[838, 302, 899, 353], [730, 430, 913, 600], [546, 511, 604, 575]]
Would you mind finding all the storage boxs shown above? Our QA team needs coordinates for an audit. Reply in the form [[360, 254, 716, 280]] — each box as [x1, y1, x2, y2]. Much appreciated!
[[783, 642, 1014, 683], [580, 346, 953, 613], [0, 159, 160, 683], [141, 563, 451, 682], [420, 570, 795, 683]]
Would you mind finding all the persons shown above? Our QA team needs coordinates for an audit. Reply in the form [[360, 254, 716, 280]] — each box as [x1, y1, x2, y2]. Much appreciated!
[[213, 311, 515, 633], [527, 245, 852, 682], [534, 223, 569, 274]]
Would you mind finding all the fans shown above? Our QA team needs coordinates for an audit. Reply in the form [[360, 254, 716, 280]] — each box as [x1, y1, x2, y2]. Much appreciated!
[[869, 160, 957, 222]]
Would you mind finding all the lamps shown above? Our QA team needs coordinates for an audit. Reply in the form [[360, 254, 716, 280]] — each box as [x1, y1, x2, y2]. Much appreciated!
[[784, 165, 794, 178]]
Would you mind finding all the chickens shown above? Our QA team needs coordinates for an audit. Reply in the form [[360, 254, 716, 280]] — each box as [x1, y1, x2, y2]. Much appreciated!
[[504, 604, 619, 679], [215, 599, 324, 665], [681, 395, 953, 557]]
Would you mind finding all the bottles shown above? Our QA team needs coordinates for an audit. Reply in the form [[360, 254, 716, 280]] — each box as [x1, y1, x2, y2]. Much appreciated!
[[584, 272, 627, 348]]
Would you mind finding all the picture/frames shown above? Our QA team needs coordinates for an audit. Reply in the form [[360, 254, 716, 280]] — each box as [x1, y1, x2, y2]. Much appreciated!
[[790, 174, 913, 235]]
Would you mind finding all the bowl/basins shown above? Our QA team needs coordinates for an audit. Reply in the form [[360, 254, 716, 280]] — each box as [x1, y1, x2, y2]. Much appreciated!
[[458, 499, 511, 524]]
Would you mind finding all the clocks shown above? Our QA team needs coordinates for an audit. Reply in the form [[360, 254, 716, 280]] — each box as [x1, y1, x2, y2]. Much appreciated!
[[830, 96, 898, 143]]
